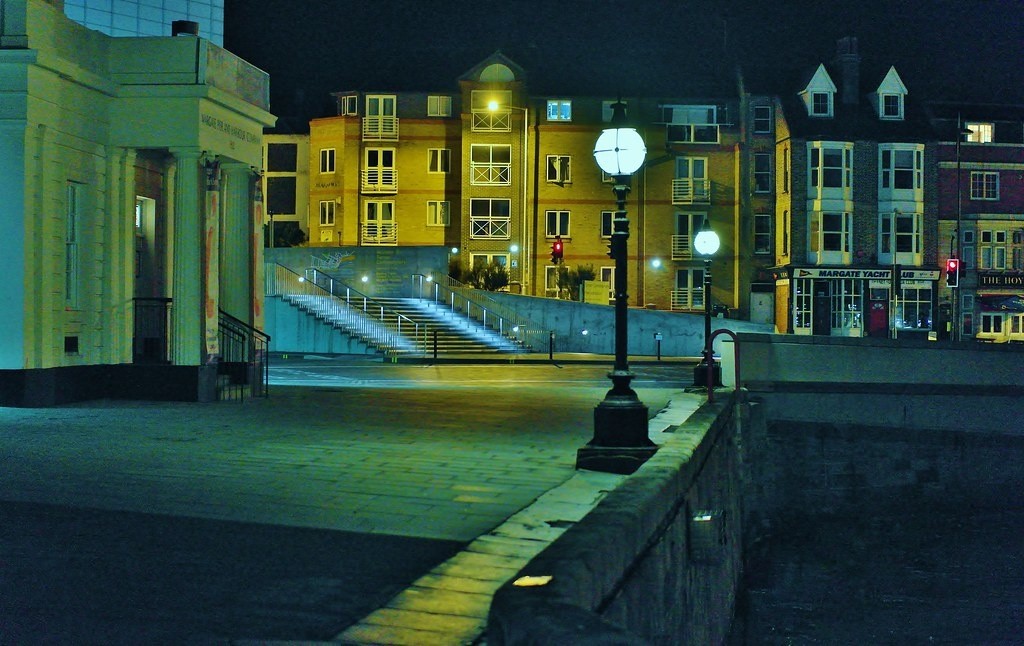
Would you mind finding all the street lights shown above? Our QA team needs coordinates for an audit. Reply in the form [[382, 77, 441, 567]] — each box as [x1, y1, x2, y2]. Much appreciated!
[[691, 220, 722, 388], [951, 129, 975, 344], [571, 97, 661, 475], [488, 101, 529, 295], [328, 89, 363, 245]]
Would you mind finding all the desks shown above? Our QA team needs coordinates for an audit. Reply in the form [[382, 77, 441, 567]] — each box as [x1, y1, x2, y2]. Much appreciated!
[[974, 338, 995, 343]]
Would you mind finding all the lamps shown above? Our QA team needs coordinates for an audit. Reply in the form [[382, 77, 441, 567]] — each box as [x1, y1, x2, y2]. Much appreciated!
[[202, 154, 220, 190]]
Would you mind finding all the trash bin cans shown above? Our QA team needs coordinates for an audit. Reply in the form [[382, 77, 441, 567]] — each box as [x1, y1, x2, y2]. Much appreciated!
[[712, 304, 729, 318]]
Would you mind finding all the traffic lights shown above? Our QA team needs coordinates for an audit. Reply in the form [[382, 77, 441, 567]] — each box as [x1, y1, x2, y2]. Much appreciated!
[[945, 258, 959, 289], [606, 231, 622, 260], [549, 245, 558, 264], [554, 242, 564, 259], [958, 259, 967, 279]]
